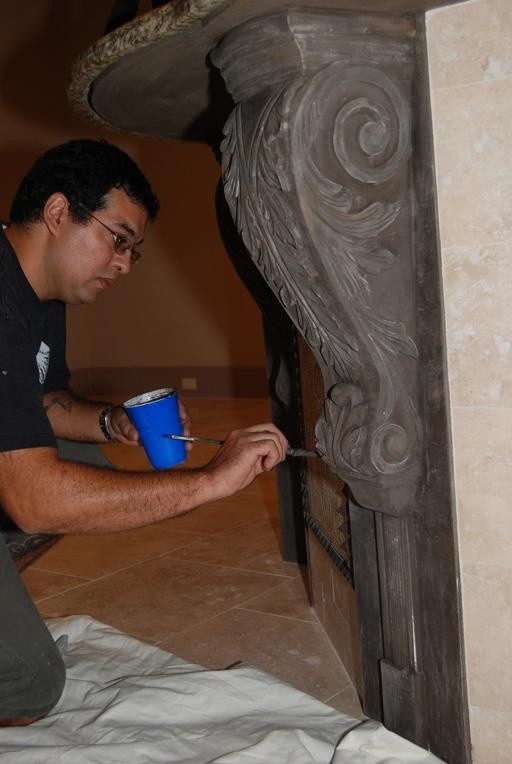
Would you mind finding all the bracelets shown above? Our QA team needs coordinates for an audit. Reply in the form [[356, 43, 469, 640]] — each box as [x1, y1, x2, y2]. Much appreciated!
[[97, 406, 119, 443]]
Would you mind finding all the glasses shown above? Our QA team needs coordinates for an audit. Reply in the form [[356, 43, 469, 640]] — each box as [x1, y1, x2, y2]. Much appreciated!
[[51, 191, 142, 267]]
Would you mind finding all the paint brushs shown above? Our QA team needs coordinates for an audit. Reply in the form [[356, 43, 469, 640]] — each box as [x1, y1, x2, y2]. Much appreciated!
[[162, 432, 320, 460]]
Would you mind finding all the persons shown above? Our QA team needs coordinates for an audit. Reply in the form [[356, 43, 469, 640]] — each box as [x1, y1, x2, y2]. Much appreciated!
[[0, 136, 290, 729]]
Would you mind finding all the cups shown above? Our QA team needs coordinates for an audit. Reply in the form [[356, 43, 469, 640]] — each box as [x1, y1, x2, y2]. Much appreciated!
[[123, 387, 187, 470]]
